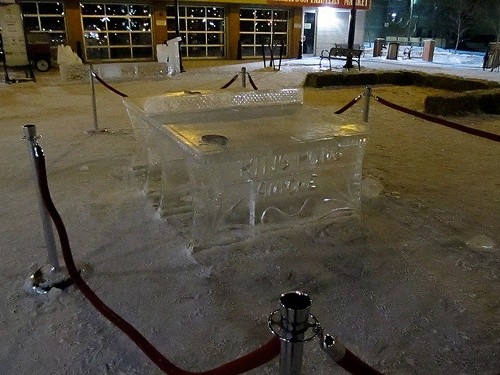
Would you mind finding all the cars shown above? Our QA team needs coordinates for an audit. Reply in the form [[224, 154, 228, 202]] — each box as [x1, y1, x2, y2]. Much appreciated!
[[0, 30, 55, 72]]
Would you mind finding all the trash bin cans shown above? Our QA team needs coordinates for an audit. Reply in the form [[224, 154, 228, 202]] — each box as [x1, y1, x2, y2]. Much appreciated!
[[373, 37, 385, 57], [386, 42, 400, 60]]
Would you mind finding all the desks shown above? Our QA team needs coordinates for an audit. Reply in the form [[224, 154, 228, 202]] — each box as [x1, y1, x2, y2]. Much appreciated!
[[121, 86, 371, 253]]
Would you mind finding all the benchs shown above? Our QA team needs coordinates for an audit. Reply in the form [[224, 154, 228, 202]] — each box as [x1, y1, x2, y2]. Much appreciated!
[[319, 46, 365, 72]]
